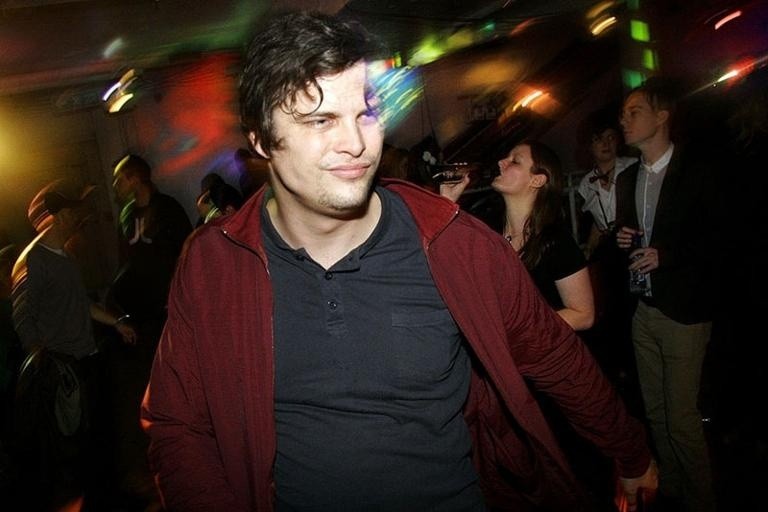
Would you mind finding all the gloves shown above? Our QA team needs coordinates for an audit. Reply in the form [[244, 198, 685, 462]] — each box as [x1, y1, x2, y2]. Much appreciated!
[[621, 457, 659, 511]]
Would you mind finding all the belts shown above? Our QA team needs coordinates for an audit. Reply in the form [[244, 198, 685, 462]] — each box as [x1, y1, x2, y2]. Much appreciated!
[[639, 295, 657, 309]]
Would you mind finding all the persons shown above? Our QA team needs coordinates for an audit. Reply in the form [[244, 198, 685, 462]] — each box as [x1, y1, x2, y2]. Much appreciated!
[[140, 11, 662, 512]]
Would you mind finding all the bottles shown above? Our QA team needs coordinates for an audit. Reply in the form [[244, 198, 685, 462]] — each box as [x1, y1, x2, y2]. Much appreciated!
[[428, 161, 499, 183], [629, 236, 649, 292]]
